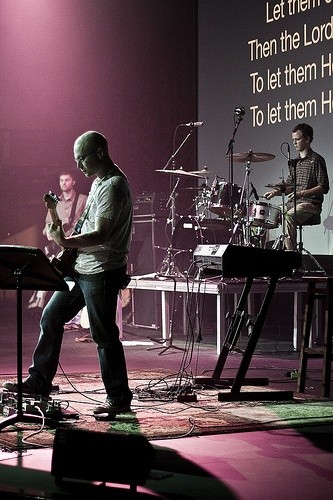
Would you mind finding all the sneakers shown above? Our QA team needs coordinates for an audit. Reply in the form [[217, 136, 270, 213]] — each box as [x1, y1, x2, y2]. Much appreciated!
[[92, 399, 130, 413], [3, 379, 50, 397]]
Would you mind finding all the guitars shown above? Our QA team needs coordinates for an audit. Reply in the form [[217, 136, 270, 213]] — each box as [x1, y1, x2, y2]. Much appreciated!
[[44, 190, 76, 277]]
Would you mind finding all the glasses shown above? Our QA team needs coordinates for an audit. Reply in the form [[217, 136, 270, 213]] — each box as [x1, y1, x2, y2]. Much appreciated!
[[75, 150, 96, 164]]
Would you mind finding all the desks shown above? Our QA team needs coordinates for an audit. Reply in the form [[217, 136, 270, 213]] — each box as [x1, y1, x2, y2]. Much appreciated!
[[121, 279, 333, 355]]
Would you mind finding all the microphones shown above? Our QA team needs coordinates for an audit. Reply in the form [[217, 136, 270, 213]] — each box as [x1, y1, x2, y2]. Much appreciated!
[[287, 145, 291, 166], [251, 183, 259, 200], [180, 121, 203, 127]]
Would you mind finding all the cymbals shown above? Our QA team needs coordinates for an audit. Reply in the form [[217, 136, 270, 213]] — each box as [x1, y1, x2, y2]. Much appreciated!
[[188, 170, 223, 173], [225, 152, 276, 162], [155, 170, 209, 179]]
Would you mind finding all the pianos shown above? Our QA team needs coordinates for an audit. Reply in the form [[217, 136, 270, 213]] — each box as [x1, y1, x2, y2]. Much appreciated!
[[191, 244, 302, 401]]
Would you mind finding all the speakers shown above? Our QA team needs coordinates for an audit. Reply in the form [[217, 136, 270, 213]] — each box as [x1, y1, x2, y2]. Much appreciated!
[[50, 428, 155, 490]]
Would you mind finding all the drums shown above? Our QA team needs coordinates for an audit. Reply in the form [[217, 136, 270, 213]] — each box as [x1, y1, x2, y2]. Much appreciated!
[[243, 201, 281, 229], [249, 228, 266, 248], [206, 181, 245, 218], [172, 215, 243, 281]]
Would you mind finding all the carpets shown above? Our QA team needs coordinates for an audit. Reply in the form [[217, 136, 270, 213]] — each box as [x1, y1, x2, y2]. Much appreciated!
[[0, 366, 333, 452]]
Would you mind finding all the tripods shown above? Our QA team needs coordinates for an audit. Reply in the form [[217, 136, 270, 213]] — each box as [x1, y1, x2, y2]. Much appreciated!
[[146, 128, 327, 355], [0, 244, 70, 431]]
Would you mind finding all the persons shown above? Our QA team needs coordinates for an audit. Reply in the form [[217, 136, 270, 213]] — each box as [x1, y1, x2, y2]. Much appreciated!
[[262, 122, 330, 251], [45, 171, 87, 260], [2, 129, 135, 415]]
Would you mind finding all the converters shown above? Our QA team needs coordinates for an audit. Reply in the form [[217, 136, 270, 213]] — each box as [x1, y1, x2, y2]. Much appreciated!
[[178, 395, 197, 402]]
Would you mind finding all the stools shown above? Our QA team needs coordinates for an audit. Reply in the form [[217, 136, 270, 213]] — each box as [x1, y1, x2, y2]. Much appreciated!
[[297, 214, 321, 255], [296, 276, 333, 398]]
